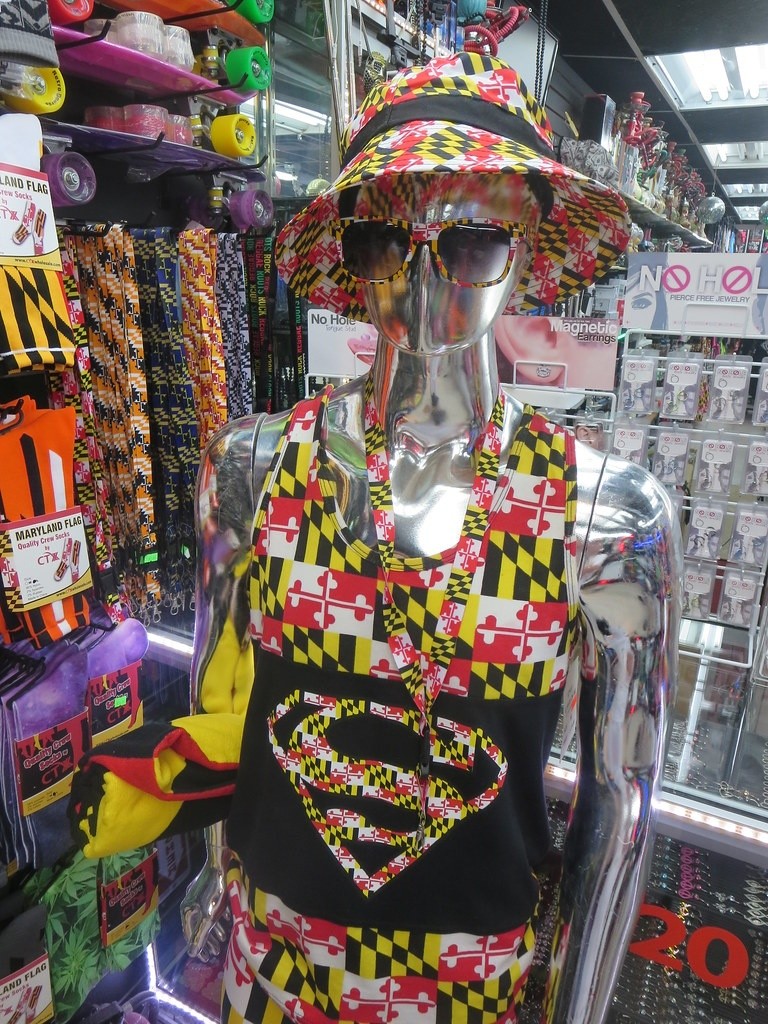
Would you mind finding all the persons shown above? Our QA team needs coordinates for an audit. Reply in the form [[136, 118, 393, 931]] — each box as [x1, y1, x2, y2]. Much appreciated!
[[68, 45, 681, 1024]]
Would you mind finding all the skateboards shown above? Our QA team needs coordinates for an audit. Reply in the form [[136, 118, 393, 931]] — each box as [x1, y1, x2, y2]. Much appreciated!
[[5, 0, 276, 232]]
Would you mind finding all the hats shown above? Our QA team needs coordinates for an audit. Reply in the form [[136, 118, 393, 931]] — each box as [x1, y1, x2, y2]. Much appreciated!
[[274, 54, 633, 326]]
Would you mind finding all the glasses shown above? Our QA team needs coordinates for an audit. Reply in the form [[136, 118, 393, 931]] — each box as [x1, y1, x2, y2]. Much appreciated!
[[327, 216, 526, 288]]
